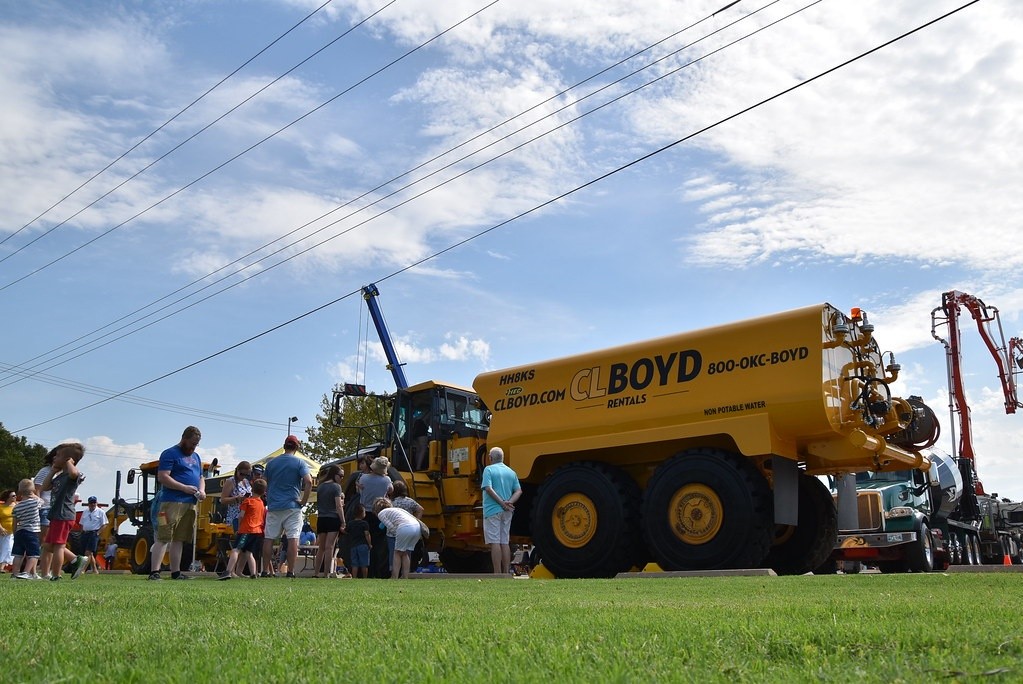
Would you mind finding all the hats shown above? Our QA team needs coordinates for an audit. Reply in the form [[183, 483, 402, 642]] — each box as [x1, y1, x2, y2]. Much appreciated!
[[370, 456, 391, 474], [252, 464, 264, 474], [237, 461, 252, 475], [285, 435, 300, 448], [88, 497, 97, 502]]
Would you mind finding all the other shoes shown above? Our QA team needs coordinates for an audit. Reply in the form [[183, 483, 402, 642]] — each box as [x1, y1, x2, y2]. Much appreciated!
[[0, 570, 6, 573], [171, 570, 189, 580], [216, 571, 296, 581], [312, 576, 319, 578], [10, 572, 52, 579], [147, 570, 163, 580]]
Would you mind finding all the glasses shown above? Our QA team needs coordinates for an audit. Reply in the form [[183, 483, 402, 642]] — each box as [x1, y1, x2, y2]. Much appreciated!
[[239, 471, 250, 477], [10, 495, 17, 498], [189, 441, 199, 447], [89, 501, 97, 504], [336, 474, 345, 479]]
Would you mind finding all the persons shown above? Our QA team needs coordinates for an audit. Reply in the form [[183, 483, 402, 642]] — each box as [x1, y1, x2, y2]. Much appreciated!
[[10, 479, 44, 580], [26, 443, 89, 581], [214, 479, 266, 581], [413, 401, 444, 470], [105, 536, 120, 570], [260, 435, 313, 578], [221, 461, 288, 579], [481, 447, 522, 573], [148, 426, 206, 580], [298, 456, 424, 579], [79, 496, 110, 570], [311, 465, 346, 578], [0, 489, 20, 573]]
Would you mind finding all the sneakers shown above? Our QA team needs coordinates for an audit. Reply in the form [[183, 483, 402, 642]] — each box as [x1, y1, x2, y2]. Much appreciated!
[[49, 575, 63, 581], [71, 555, 88, 580]]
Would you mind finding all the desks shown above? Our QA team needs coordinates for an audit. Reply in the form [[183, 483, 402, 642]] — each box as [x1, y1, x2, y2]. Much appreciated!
[[297, 545, 321, 570]]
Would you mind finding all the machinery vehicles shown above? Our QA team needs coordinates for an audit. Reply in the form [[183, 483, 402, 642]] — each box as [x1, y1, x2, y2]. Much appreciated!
[[813, 444, 983, 573], [931, 289, 1022, 563], [63, 458, 260, 576]]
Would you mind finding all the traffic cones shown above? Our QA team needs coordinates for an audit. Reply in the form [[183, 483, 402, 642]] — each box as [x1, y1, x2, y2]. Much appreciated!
[[1003, 555, 1012, 566]]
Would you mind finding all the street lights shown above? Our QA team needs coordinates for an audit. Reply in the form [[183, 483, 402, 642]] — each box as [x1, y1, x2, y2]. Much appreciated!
[[287, 416, 298, 436]]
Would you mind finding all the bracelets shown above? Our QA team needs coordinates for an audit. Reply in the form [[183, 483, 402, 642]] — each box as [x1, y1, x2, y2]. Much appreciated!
[[236, 495, 238, 501]]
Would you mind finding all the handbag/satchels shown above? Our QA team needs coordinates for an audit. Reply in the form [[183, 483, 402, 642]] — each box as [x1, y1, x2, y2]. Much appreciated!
[[416, 518, 430, 540]]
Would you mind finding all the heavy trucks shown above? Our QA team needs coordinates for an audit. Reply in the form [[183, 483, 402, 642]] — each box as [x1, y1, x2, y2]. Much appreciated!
[[307, 302, 940, 577]]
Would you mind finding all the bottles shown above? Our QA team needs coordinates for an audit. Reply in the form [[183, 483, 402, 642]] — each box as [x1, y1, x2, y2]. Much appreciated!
[[194, 490, 203, 503], [160, 511, 167, 526]]
